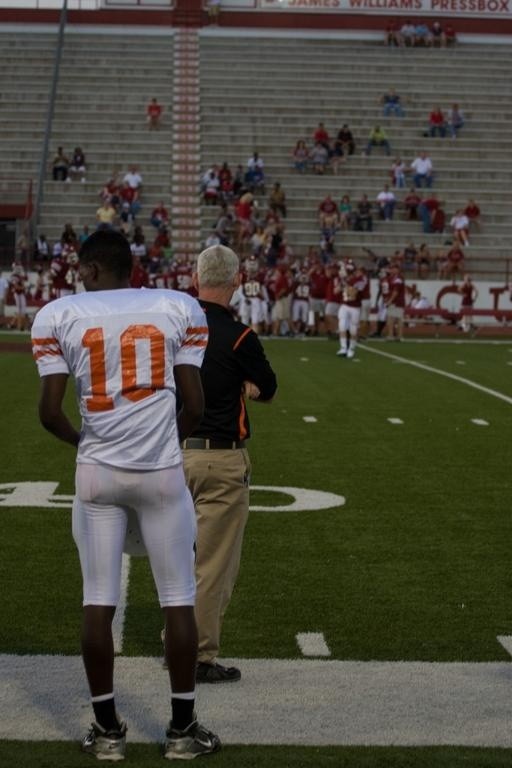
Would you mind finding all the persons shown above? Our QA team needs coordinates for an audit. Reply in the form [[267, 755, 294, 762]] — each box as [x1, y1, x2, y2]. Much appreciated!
[[30, 229, 222, 760], [415, 23, 429, 45], [400, 20, 417, 47], [445, 24, 456, 45], [386, 19, 400, 47], [161, 245, 277, 683], [428, 21, 446, 48], [1, 78, 511, 341], [335, 259, 365, 358]]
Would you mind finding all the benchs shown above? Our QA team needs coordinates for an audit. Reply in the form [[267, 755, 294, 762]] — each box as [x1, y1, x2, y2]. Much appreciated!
[[0, 38, 509, 341]]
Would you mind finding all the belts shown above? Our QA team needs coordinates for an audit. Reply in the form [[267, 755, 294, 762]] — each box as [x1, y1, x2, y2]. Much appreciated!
[[182, 440, 244, 449]]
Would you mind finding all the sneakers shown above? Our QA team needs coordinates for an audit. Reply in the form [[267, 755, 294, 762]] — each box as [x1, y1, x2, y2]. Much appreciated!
[[196, 662, 240, 683], [165, 720, 220, 760], [81, 722, 127, 762]]
